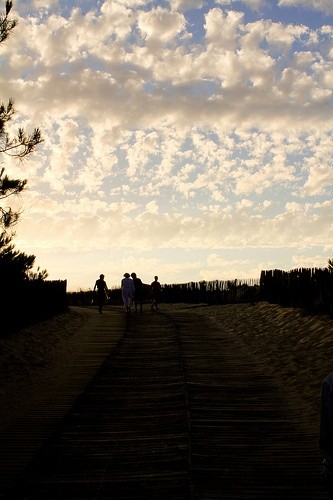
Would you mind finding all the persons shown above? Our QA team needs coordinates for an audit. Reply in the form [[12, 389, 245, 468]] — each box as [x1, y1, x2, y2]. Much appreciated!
[[121, 272, 135, 313], [93, 274, 109, 314], [150, 276, 162, 311], [130, 272, 146, 313]]
